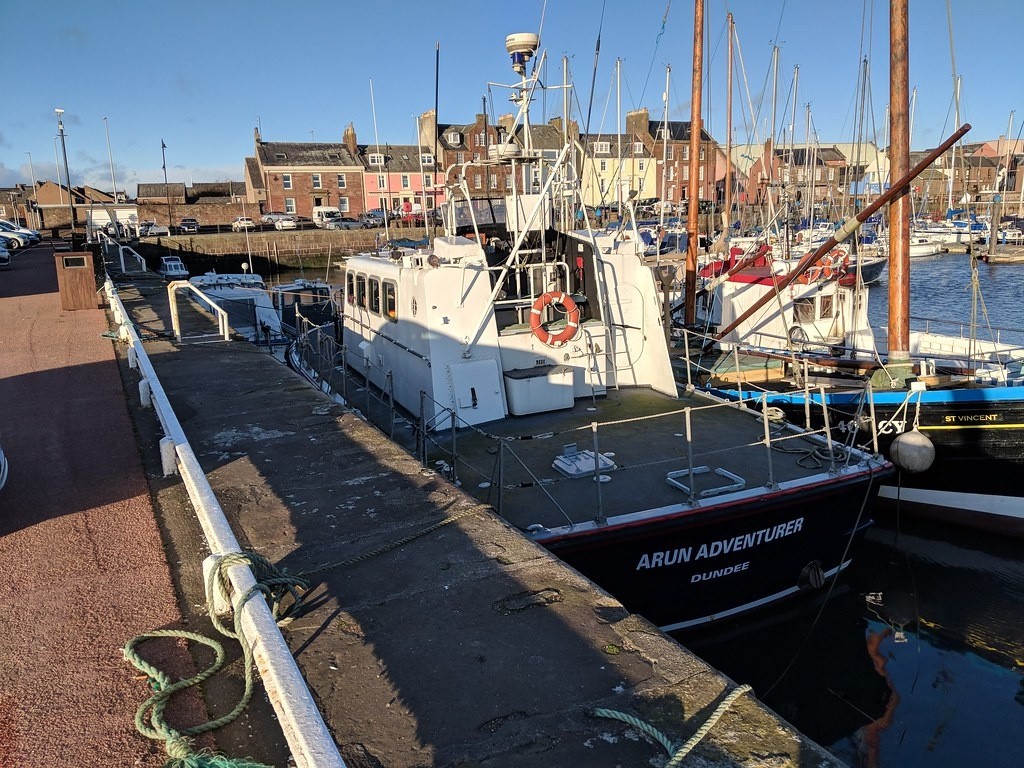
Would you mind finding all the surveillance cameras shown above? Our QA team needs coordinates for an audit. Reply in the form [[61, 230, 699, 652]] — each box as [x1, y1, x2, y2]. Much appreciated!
[[55, 109, 65, 116]]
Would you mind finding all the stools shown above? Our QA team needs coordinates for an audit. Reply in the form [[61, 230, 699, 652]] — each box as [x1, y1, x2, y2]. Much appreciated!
[[502, 364, 575, 416]]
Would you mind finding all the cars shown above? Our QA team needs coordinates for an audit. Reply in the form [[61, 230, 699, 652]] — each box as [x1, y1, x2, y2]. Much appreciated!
[[107, 198, 508, 237], [0, 217, 43, 266]]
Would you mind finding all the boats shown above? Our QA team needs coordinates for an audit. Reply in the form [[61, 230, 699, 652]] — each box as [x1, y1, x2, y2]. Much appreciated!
[[158, 240, 189, 282], [242, 197, 340, 338], [287, 1, 898, 650], [189, 262, 287, 367]]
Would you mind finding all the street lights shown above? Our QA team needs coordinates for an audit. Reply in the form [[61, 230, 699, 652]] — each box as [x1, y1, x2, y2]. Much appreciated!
[[102, 116, 119, 204], [25, 151, 40, 229], [55, 108, 78, 232], [160, 138, 174, 235], [54, 137, 64, 206]]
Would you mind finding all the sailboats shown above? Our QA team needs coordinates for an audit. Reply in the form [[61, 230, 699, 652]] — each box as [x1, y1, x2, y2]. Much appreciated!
[[551, 12, 1023, 321], [640, 0, 1023, 544]]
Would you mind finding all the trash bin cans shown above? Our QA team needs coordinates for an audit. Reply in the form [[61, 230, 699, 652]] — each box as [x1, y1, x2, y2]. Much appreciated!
[[53, 251, 98, 310]]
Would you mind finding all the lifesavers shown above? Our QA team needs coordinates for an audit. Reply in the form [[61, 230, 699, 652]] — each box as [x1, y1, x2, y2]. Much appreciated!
[[797, 253, 823, 285], [529, 291, 580, 347], [823, 250, 849, 280]]
